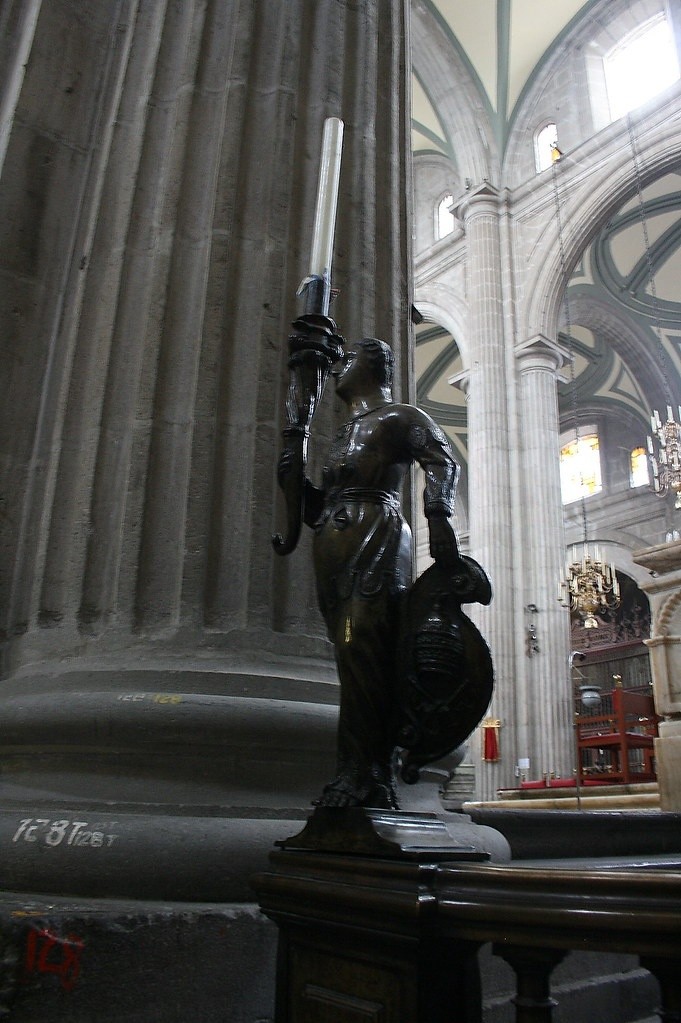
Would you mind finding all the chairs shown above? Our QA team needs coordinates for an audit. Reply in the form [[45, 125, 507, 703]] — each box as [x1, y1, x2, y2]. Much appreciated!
[[575, 673, 659, 788]]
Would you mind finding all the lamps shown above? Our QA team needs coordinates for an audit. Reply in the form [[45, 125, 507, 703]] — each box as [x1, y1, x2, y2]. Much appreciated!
[[552, 143, 624, 631], [624, 112, 681, 510], [568, 651, 602, 708]]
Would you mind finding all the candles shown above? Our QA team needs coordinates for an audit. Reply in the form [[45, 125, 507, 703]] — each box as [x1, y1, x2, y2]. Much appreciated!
[[295, 114, 345, 326]]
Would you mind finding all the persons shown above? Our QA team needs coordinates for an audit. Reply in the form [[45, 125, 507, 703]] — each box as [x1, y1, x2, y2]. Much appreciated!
[[275, 337, 462, 845]]
